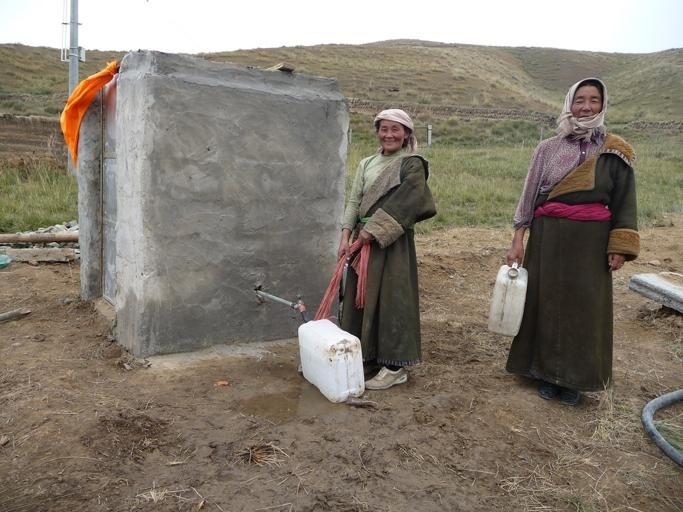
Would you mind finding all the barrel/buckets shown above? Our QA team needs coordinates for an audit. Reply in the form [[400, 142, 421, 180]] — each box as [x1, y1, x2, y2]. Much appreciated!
[[298, 319, 365, 402], [487, 260, 529, 336]]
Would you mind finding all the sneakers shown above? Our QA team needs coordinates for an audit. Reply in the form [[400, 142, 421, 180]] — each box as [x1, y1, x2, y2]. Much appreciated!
[[536, 380, 581, 404], [365, 365, 407, 390]]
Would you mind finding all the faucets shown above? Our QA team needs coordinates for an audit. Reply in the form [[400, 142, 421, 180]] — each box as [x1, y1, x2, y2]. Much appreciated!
[[294, 295, 305, 314]]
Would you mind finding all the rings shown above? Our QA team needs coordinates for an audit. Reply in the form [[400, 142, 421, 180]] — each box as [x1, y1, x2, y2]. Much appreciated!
[[619, 263, 623, 266]]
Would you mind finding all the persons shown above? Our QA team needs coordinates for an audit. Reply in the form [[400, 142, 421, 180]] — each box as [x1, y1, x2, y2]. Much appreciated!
[[506, 78, 639, 407], [335, 107, 436, 389]]
